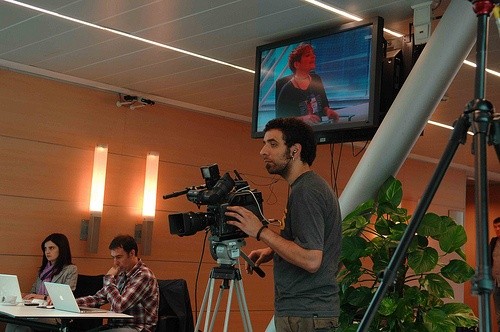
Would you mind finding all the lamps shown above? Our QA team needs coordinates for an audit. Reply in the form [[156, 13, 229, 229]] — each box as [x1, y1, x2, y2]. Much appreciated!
[[80, 144, 109, 253], [134, 152, 159, 256]]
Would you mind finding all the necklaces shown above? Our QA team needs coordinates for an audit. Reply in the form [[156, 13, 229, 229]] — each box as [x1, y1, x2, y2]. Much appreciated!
[[294, 75, 312, 99]]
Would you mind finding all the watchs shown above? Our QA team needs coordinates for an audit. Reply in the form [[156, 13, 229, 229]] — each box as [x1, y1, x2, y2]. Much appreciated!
[[44, 294, 47, 300]]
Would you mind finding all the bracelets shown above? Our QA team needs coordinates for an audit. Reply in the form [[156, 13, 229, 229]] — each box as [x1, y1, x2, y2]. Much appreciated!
[[257, 225, 268, 241]]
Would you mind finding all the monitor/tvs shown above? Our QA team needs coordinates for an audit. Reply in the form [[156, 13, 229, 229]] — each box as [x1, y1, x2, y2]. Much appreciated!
[[251, 16, 384, 146]]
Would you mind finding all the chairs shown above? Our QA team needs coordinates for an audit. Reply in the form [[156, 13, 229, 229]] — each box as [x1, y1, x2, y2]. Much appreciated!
[[62, 274, 194, 332]]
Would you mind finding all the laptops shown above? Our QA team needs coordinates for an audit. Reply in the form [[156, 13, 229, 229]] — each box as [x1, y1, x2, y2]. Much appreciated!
[[43, 282, 108, 314], [0, 274, 32, 306]]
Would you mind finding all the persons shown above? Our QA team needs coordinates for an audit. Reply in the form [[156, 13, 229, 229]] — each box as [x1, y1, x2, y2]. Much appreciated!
[[47, 234, 159, 332], [276, 44, 340, 125], [225, 117, 342, 332], [489, 217, 500, 332], [5, 233, 77, 332]]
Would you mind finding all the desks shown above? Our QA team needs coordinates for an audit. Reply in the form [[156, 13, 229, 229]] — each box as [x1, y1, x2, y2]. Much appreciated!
[[0, 303, 135, 332]]
